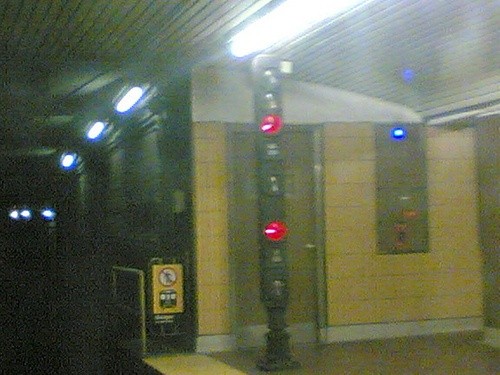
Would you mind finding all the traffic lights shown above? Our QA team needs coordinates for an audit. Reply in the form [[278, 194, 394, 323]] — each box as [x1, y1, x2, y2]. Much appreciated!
[[253, 53, 285, 195], [258, 195, 289, 306]]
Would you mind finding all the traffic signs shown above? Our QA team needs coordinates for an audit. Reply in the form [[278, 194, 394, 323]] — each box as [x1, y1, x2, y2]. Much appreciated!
[[152, 264, 184, 313]]
